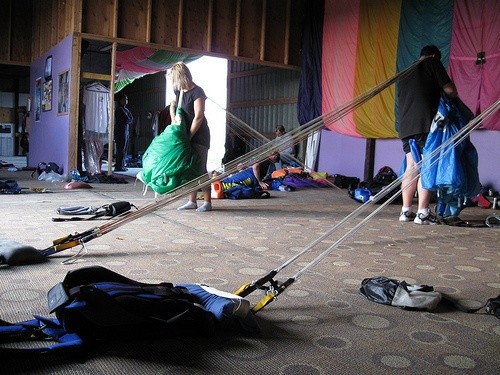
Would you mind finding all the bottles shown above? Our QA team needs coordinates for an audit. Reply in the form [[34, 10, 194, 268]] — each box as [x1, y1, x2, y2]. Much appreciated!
[[210, 174, 225, 199], [493, 197, 498, 209], [489, 188, 493, 196], [354, 188, 374, 203], [72, 168, 77, 182]]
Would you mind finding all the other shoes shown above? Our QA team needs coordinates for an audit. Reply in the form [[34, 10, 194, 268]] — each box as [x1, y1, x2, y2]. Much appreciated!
[[114, 168, 127, 172]]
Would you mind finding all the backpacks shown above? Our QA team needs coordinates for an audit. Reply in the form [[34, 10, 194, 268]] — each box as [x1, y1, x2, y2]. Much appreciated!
[[48, 266, 218, 344], [359, 277, 442, 311], [224, 184, 270, 201]]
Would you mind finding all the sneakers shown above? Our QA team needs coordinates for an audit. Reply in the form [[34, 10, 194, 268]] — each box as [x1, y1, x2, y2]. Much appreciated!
[[414, 210, 440, 225], [398, 210, 416, 221]]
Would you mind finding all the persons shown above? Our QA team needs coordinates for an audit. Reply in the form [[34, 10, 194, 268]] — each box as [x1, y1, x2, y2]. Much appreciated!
[[273, 124, 295, 170], [18, 113, 30, 154], [212, 170, 222, 175], [253, 151, 281, 189], [394, 45, 457, 225], [59, 82, 68, 112], [114, 94, 133, 171], [237, 163, 243, 172], [170, 63, 211, 212]]
